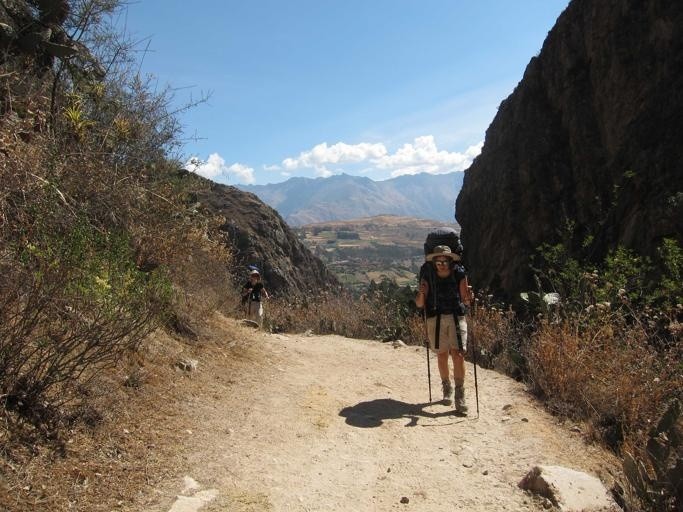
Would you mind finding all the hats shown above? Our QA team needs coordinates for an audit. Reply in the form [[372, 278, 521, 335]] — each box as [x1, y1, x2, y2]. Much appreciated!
[[249, 270, 261, 280], [427, 245, 461, 262]]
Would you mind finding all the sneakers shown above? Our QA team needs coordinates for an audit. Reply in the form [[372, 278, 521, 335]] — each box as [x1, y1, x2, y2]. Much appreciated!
[[454, 397, 468, 411], [443, 386, 453, 405]]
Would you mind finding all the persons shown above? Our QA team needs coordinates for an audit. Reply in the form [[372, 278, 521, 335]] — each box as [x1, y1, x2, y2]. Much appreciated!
[[241, 270, 269, 330], [414, 245, 475, 413]]
